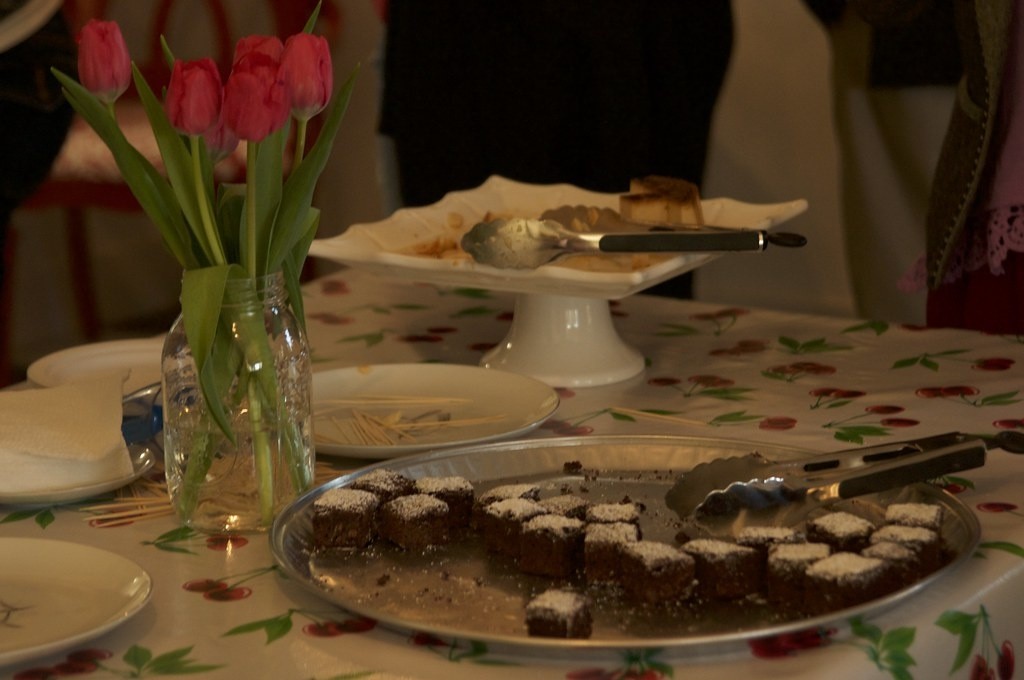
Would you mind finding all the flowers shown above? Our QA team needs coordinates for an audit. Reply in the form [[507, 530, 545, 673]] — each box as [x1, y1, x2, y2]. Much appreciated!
[[57, 13, 358, 271]]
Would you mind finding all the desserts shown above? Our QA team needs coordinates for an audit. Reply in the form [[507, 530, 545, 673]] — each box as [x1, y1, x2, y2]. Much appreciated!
[[314, 472, 942, 642], [622, 173, 706, 228]]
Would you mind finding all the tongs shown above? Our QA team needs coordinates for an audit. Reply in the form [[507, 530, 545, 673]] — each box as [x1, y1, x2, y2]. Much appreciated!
[[662, 430, 989, 536], [454, 201, 808, 272]]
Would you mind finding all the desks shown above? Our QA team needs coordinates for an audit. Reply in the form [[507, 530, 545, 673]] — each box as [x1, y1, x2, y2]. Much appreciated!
[[0, 279, 1024, 680]]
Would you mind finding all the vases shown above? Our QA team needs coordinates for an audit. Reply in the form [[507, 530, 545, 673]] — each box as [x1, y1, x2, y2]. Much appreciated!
[[159, 282, 318, 541]]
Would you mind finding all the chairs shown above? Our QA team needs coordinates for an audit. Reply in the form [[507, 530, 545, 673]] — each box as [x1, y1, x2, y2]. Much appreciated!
[[48, 0, 385, 341]]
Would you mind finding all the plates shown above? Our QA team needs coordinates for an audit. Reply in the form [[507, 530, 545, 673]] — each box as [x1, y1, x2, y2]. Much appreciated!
[[307, 175, 809, 390], [25, 338, 171, 403], [0, 536, 153, 667], [289, 362, 562, 459], [266, 434, 979, 654], [0, 443, 156, 509]]
